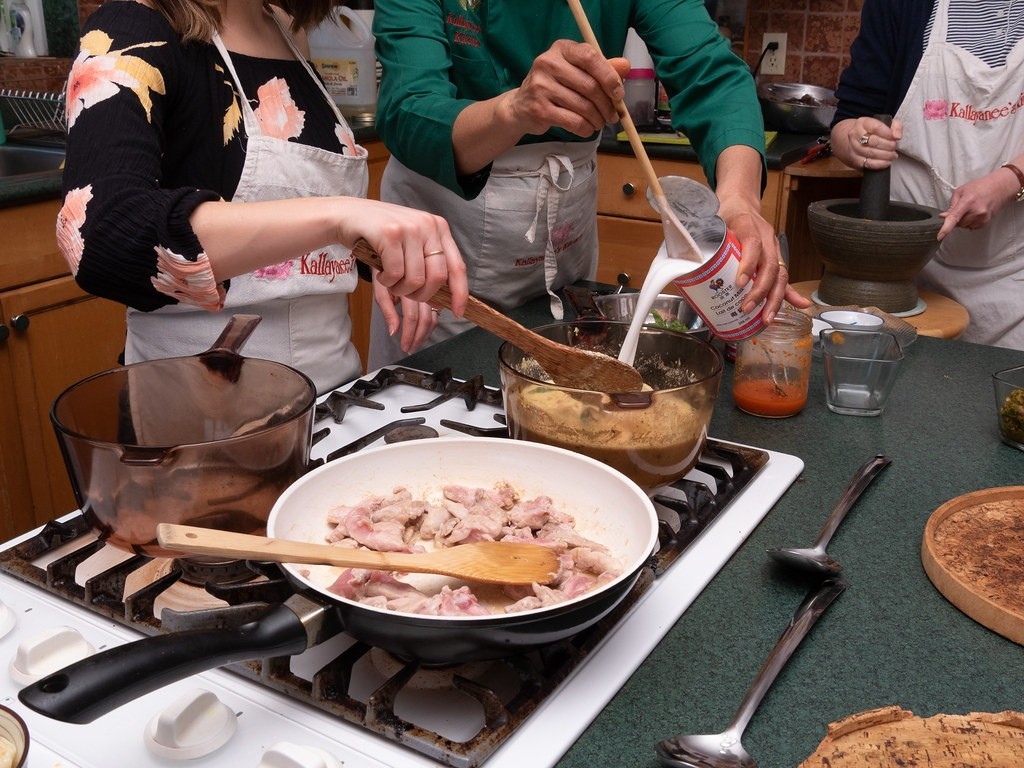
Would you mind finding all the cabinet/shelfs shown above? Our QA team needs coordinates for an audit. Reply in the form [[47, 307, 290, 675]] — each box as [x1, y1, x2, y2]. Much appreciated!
[[1, 150, 829, 542]]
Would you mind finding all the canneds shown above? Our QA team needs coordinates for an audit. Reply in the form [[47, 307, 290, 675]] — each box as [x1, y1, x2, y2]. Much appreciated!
[[647, 175, 775, 341], [724, 305, 813, 419]]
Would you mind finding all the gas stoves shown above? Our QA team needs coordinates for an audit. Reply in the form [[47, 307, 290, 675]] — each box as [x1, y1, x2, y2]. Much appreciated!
[[0, 365, 805, 768]]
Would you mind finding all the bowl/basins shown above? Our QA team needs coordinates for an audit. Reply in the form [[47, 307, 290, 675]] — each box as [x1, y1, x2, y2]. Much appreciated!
[[0, 704, 30, 768], [820, 311, 884, 332], [759, 82, 840, 129], [577, 293, 712, 344], [811, 317, 833, 343]]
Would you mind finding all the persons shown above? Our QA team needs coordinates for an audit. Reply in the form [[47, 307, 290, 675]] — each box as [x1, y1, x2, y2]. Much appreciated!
[[829, 0, 1024, 349], [56, 0, 470, 447], [367, 0, 812, 375]]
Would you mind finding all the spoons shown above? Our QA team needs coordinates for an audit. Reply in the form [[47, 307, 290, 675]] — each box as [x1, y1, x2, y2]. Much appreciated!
[[767, 454, 892, 573], [656, 579, 847, 768]]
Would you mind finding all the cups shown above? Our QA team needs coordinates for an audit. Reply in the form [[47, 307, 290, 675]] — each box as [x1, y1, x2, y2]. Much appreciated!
[[819, 329, 904, 417], [993, 366, 1024, 450]]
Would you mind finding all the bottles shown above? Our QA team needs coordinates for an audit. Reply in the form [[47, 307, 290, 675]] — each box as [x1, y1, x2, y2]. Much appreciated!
[[658, 80, 671, 116], [307, 6, 377, 106], [734, 308, 813, 419], [5, 0, 38, 60], [623, 69, 655, 128]]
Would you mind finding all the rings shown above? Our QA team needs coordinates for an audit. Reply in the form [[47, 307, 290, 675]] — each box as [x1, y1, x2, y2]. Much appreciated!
[[779, 261, 788, 270], [424, 250, 443, 258], [863, 157, 867, 167], [860, 133, 872, 145], [432, 308, 440, 315]]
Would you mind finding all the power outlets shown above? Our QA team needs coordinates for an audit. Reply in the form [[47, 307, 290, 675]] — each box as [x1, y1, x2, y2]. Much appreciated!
[[759, 32, 787, 75]]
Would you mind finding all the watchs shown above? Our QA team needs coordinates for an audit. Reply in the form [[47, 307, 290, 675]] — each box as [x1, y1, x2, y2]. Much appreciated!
[[1001, 164, 1024, 202]]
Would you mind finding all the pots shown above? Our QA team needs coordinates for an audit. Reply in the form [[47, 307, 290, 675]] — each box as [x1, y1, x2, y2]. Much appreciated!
[[498, 321, 724, 490], [17, 437, 659, 724], [50, 314, 317, 559]]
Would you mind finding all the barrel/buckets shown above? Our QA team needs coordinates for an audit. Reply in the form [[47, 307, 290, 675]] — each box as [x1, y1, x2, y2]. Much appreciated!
[[647, 176, 769, 342]]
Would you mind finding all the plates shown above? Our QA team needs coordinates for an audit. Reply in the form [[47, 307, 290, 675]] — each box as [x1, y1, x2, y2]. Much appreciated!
[[657, 117, 671, 126], [789, 304, 917, 349]]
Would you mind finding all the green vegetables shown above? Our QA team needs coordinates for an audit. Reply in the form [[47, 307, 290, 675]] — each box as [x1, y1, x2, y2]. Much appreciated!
[[647, 308, 689, 331]]
